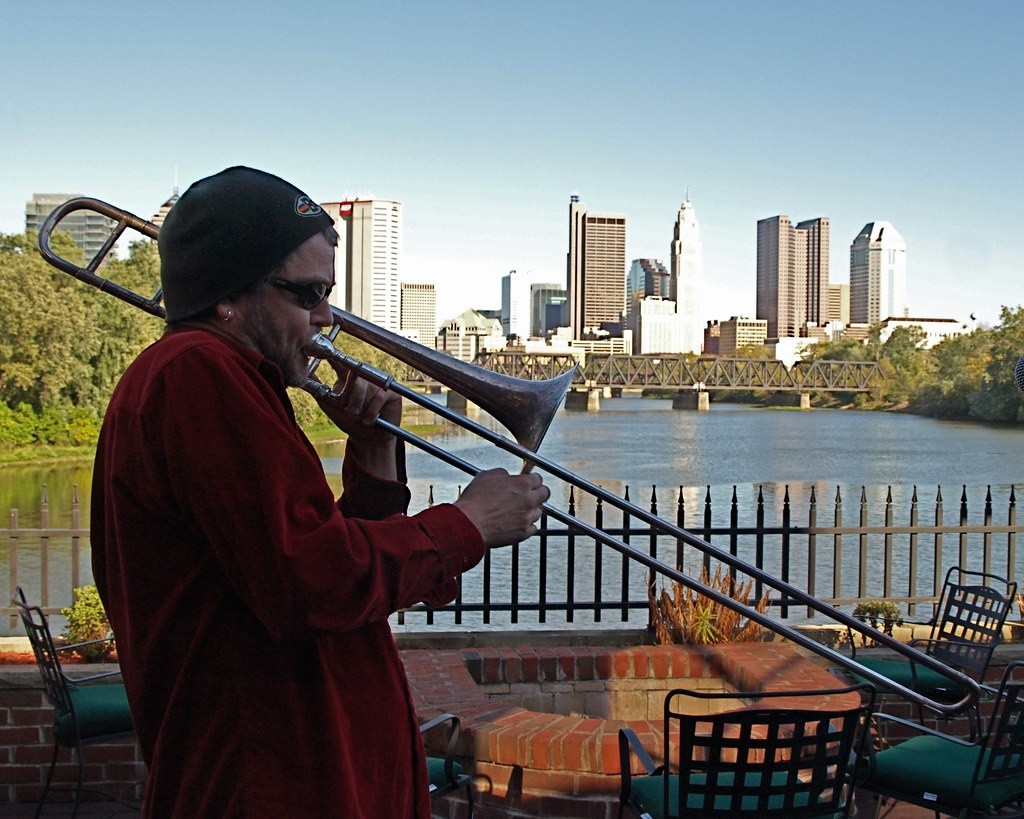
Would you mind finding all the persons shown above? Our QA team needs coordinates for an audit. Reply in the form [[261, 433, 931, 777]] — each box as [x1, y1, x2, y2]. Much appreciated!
[[87, 164, 553, 818]]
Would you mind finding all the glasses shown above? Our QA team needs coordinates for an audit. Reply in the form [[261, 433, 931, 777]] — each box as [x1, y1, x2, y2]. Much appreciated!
[[263, 274, 332, 310]]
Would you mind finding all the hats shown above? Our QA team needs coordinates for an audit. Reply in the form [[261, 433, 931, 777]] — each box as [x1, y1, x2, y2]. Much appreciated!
[[157, 164, 335, 323]]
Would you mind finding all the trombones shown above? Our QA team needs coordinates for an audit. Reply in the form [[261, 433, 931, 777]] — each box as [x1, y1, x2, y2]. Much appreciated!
[[37, 194, 983, 717]]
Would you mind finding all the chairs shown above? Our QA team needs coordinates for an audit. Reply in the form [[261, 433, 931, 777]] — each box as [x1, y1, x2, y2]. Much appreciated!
[[849, 566, 1018, 746], [9, 585, 145, 819], [845, 661, 1024, 819], [617, 682, 877, 819], [418, 713, 473, 819]]
